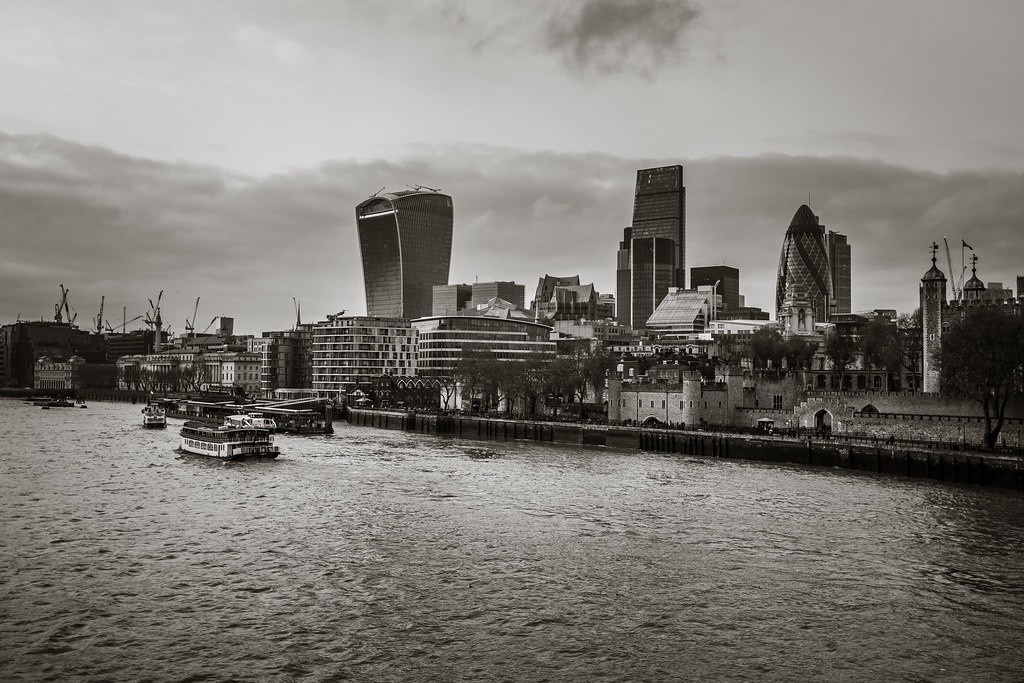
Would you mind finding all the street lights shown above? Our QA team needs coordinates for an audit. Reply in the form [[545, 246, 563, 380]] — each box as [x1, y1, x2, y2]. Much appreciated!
[[658, 377, 670, 429], [629, 381, 650, 429]]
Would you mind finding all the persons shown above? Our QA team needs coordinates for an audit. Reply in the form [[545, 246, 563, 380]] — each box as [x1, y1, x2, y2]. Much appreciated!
[[733, 426, 773, 436], [873, 434, 876, 443], [653, 420, 685, 430], [623, 418, 636, 427], [816, 429, 831, 440], [788, 427, 796, 438], [1002, 438, 1006, 446], [890, 434, 895, 445], [587, 417, 592, 423], [562, 414, 566, 421]]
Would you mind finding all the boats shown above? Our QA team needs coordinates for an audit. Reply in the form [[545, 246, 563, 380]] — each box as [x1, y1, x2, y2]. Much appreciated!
[[178, 412, 281, 461], [33, 400, 75, 408], [142, 403, 167, 428]]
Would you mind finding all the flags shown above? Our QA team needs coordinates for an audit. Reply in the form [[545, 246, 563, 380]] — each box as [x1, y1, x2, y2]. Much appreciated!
[[963, 242, 973, 250]]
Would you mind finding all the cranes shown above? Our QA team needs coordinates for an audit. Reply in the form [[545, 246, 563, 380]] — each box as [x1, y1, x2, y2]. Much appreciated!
[[92, 294, 106, 334], [53, 282, 78, 327], [944, 235, 964, 301], [104, 315, 143, 333], [185, 295, 201, 333], [142, 289, 164, 330]]
